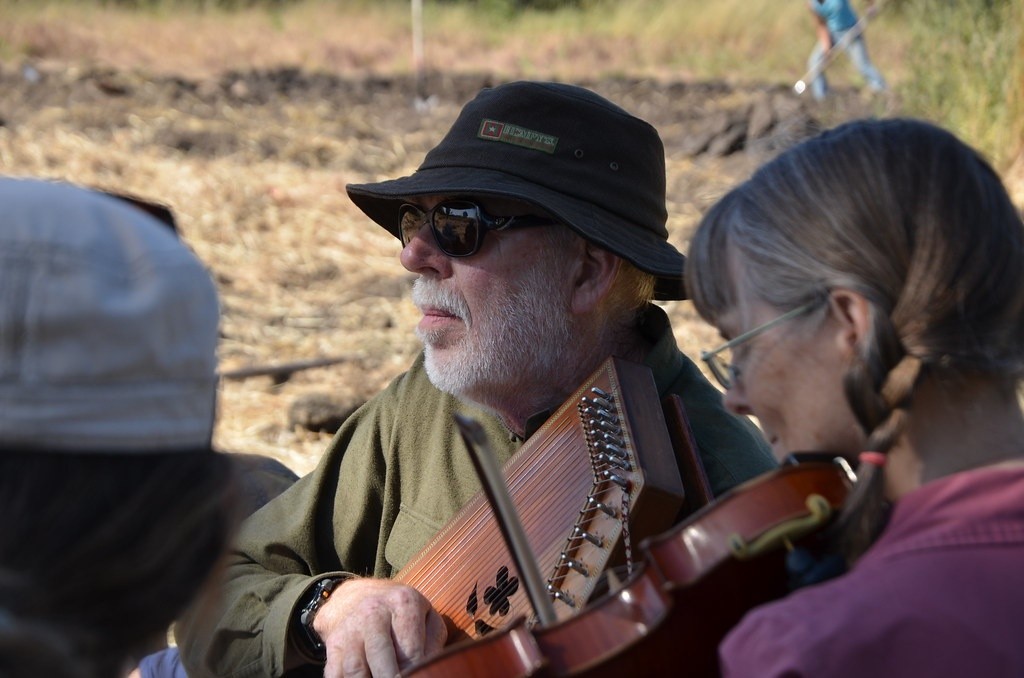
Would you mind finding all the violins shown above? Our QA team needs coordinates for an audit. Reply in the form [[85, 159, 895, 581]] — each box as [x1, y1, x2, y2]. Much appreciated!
[[386, 434, 912, 678]]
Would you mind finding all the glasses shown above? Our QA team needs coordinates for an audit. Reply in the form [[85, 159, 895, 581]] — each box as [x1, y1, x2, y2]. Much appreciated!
[[701, 294, 832, 389], [399, 198, 564, 257]]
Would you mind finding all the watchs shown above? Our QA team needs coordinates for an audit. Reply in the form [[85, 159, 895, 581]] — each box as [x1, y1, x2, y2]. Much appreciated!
[[292, 573, 352, 667]]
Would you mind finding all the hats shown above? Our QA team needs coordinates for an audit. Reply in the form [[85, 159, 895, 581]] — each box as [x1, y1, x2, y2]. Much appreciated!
[[0, 177, 220, 453], [345, 83, 695, 301]]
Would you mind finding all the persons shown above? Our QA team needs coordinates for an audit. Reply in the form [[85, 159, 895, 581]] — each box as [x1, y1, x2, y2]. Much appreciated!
[[806, 0, 886, 100], [166, 79, 779, 678], [684, 117, 1024, 678], [0, 175, 299, 678]]
